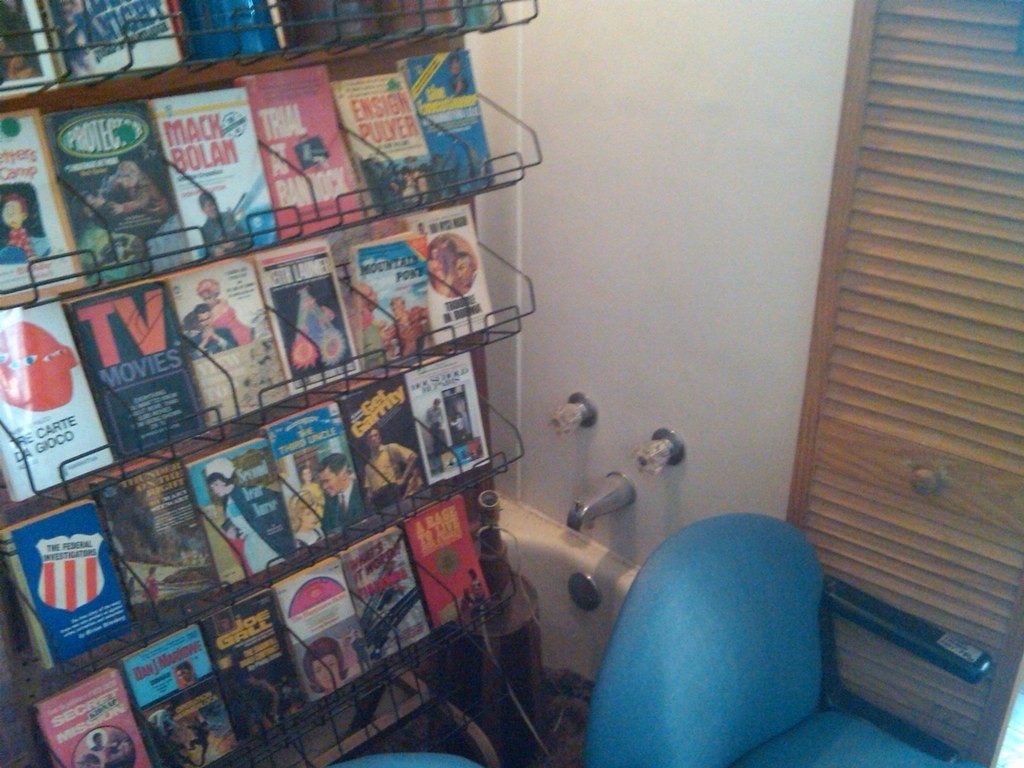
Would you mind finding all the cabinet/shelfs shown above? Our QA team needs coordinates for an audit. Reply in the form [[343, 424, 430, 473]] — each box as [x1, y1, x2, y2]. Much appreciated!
[[0, 0, 540, 768]]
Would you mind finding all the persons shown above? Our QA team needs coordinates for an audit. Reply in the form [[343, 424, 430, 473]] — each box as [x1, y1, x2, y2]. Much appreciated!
[[456, 397, 470, 433], [362, 426, 419, 507], [427, 399, 447, 452], [146, 704, 181, 738], [447, 412, 467, 437], [186, 715, 211, 763], [176, 661, 196, 687], [190, 192, 248, 257]]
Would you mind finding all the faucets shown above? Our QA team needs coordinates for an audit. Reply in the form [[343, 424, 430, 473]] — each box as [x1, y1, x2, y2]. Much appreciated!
[[564, 468, 640, 535]]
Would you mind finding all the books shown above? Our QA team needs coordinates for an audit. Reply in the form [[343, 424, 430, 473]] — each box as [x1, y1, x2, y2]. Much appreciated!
[[0, 0, 509, 768]]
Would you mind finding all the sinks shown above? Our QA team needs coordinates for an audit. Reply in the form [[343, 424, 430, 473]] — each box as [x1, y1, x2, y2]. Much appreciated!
[[494, 492, 642, 686]]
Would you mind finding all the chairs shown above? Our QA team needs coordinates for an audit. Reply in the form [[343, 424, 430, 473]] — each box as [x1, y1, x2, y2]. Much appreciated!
[[584, 514, 991, 768]]
